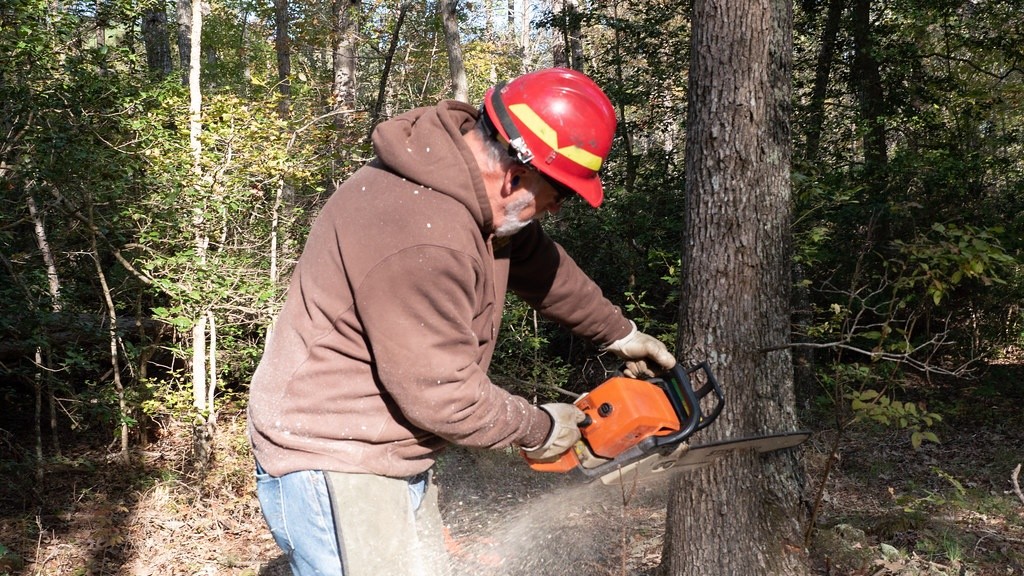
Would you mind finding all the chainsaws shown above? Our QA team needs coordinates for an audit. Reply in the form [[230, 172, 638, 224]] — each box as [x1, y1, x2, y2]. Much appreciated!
[[521, 349, 815, 486]]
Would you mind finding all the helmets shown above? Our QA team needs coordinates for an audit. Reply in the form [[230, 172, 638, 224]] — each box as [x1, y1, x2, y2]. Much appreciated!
[[486, 68, 618, 208]]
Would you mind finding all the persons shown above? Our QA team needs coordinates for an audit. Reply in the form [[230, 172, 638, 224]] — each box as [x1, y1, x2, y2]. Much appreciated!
[[244, 68, 679, 576]]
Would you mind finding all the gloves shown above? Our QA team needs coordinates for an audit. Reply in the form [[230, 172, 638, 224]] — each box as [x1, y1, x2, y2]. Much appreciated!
[[520, 404, 585, 463], [607, 320, 675, 379]]
